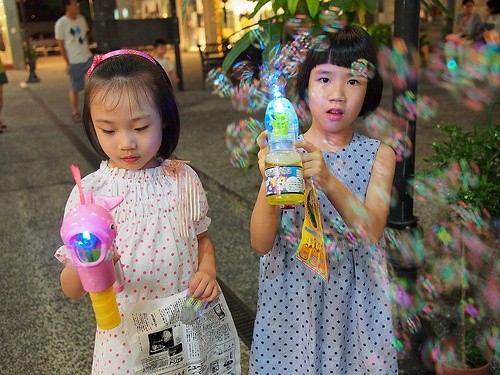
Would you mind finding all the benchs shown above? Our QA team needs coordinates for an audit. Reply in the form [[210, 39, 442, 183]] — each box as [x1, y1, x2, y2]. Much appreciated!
[[198, 42, 237, 91]]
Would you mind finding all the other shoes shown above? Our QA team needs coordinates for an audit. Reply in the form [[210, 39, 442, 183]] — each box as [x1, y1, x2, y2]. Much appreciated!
[[0, 119, 6, 128], [72, 112, 82, 123]]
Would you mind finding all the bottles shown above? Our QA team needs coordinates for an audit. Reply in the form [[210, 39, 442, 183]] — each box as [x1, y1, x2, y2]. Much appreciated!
[[264, 86, 305, 205]]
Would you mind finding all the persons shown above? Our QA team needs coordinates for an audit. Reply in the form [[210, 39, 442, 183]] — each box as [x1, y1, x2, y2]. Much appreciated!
[[54, 49, 218, 375], [54, 0, 95, 122], [248, 24, 397, 374], [0, 29, 8, 134]]
